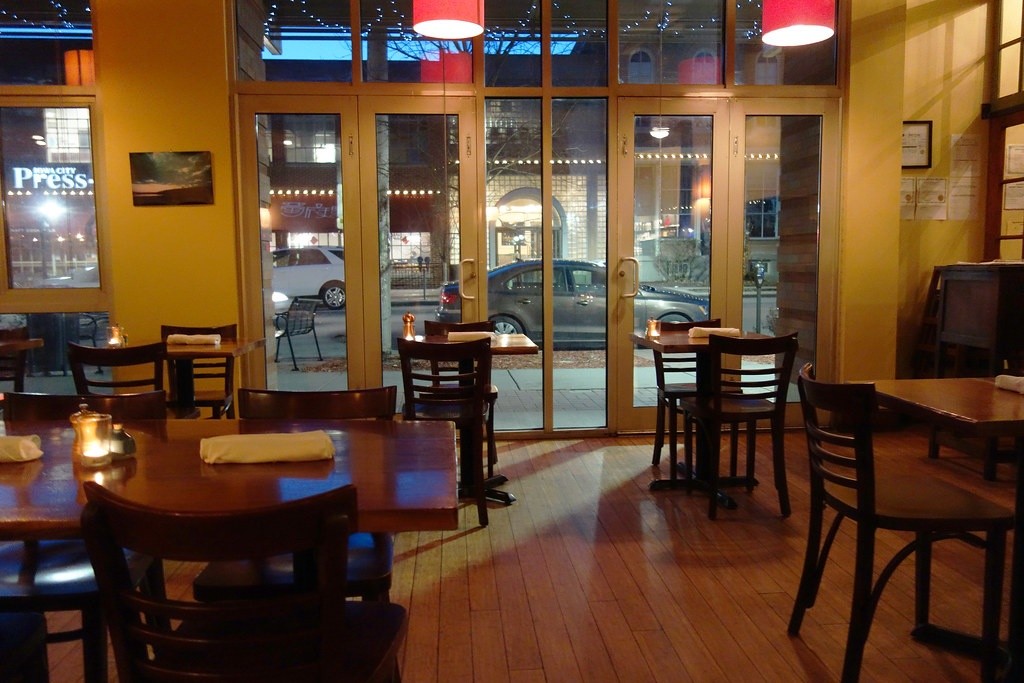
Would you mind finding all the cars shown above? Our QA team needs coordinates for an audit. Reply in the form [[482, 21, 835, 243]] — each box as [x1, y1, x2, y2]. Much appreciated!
[[268, 244, 347, 311], [40, 264, 99, 288], [434, 259, 711, 354]]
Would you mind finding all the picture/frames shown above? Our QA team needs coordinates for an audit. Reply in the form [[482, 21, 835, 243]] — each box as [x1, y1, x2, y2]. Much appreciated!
[[901, 120, 932, 170], [129, 150, 214, 207]]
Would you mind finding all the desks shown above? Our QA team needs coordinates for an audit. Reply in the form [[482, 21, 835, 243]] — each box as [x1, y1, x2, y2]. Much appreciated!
[[398, 332, 540, 506], [0, 338, 45, 353], [627, 330, 775, 511], [162, 336, 268, 420], [0, 419, 461, 683], [845, 376, 1024, 683]]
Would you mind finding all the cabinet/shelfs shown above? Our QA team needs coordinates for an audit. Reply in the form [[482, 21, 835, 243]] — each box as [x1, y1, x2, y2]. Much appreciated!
[[924, 264, 1024, 482]]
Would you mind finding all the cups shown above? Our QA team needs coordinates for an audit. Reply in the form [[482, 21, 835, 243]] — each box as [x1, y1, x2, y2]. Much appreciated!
[[648, 320, 661, 340], [77, 414, 113, 467], [107, 327, 121, 347]]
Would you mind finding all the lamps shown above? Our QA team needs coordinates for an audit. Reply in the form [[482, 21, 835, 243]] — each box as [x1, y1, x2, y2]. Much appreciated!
[[412, 0, 485, 40], [419, 49, 472, 83], [679, 56, 724, 85], [762, 0, 836, 47]]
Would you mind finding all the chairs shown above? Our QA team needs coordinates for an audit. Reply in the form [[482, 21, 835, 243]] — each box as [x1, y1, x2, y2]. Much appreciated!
[[425, 319, 497, 466], [161, 323, 238, 419], [652, 318, 743, 466], [65, 338, 168, 395], [0, 327, 30, 393], [795, 363, 1016, 683], [273, 297, 323, 372], [79, 482, 408, 683], [187, 385, 399, 605], [0, 389, 172, 683], [396, 337, 492, 529], [675, 331, 802, 523], [0, 609, 50, 683]]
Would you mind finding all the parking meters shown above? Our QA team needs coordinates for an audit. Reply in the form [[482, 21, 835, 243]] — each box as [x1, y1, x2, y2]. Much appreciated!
[[755, 261, 766, 334], [418, 257, 430, 301]]
